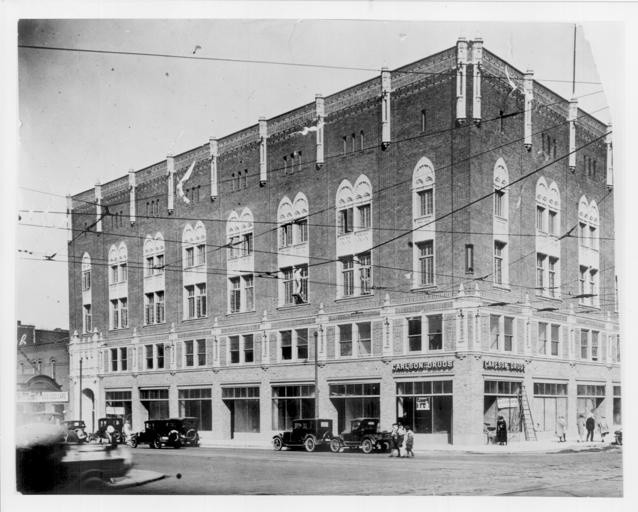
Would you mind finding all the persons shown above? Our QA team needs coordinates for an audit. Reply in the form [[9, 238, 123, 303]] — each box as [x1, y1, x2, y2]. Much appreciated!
[[558, 415, 567, 442], [405, 425, 415, 458], [388, 423, 405, 457], [105, 425, 117, 450], [577, 415, 585, 443], [496, 415, 507, 445], [123, 420, 132, 446], [597, 415, 610, 444], [586, 416, 595, 442], [396, 425, 406, 446]]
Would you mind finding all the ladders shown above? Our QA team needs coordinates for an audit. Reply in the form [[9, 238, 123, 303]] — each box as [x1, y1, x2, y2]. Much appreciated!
[[517, 385, 537, 441]]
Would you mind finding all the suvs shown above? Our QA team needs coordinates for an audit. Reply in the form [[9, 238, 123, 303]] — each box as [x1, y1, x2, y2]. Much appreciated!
[[17, 423, 133, 493], [59, 416, 200, 448], [272, 417, 333, 450], [330, 416, 395, 454]]
